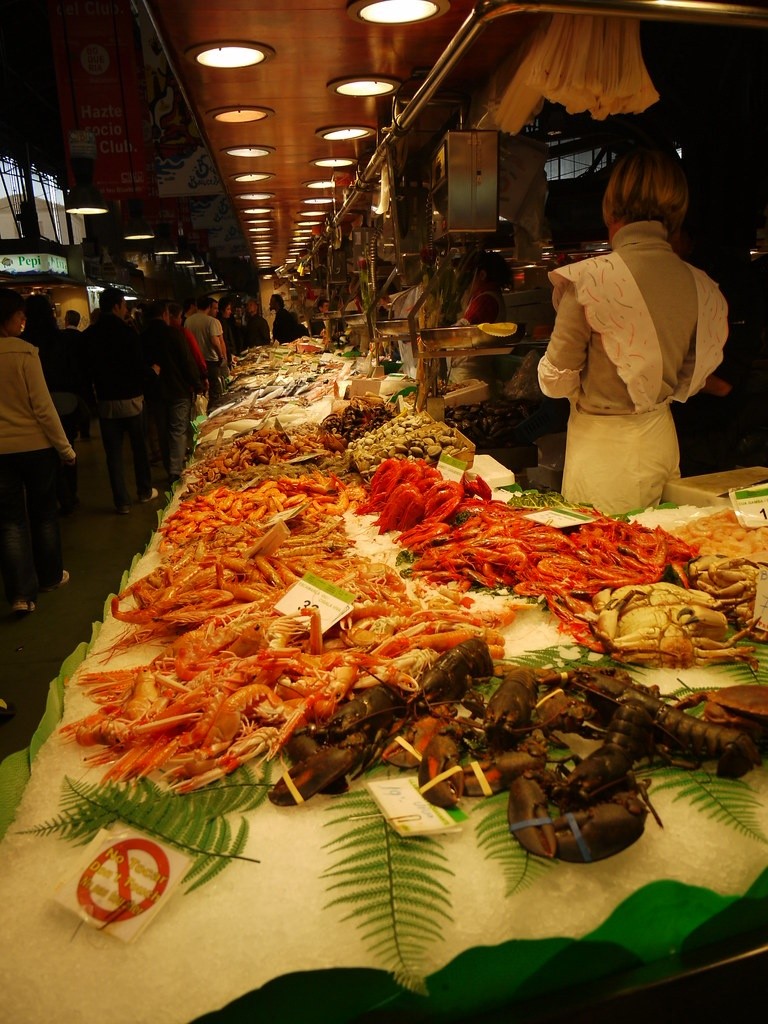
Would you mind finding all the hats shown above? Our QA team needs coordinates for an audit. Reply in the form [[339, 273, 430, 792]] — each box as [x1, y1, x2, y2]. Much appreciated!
[[460, 249, 487, 271]]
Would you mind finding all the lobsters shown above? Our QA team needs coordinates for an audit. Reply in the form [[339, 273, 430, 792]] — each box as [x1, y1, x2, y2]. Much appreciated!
[[264, 632, 768, 867]]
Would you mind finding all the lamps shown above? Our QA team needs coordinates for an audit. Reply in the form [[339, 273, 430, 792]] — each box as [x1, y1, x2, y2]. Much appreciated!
[[154, 177, 179, 255], [109, 0, 156, 241], [60, 0, 110, 216], [173, 237, 230, 290]]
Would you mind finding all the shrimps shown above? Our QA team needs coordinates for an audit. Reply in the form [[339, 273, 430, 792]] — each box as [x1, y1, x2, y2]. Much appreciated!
[[56, 455, 768, 796]]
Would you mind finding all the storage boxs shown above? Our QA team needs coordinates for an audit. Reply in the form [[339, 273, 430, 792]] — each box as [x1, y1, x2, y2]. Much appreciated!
[[352, 410, 476, 479]]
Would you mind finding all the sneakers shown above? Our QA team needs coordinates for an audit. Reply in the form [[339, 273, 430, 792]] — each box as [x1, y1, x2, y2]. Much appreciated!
[[39, 571, 70, 591], [116, 504, 130, 513], [10, 601, 35, 613], [138, 488, 158, 502]]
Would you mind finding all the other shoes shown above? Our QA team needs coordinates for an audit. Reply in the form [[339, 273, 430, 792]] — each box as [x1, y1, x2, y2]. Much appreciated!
[[64, 496, 81, 518], [186, 447, 193, 454], [167, 474, 180, 483], [167, 456, 190, 465]]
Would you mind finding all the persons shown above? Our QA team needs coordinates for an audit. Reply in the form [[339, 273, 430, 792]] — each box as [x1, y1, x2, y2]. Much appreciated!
[[377, 279, 401, 360], [453, 252, 514, 377], [309, 299, 344, 335], [0, 287, 76, 614], [537, 147, 729, 516], [17, 288, 270, 517], [270, 295, 310, 347]]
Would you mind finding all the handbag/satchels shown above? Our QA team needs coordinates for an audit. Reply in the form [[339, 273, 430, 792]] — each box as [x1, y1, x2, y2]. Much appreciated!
[[194, 394, 208, 417]]
